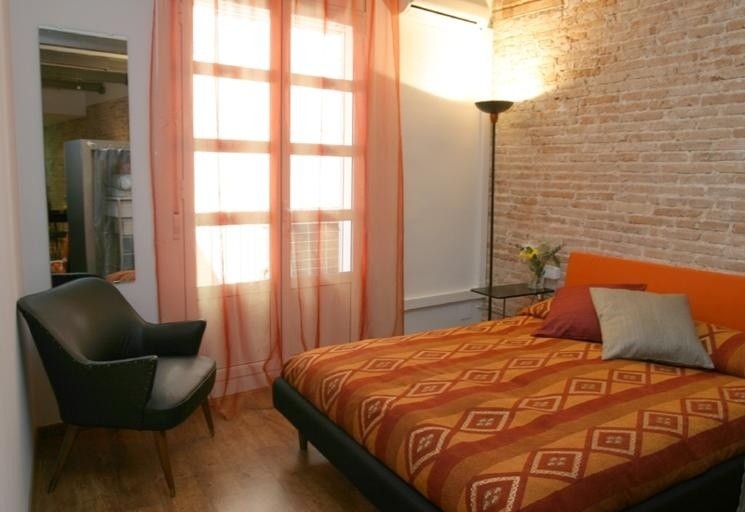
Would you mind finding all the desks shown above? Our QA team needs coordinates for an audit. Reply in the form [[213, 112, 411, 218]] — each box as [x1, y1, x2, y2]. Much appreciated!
[[471, 283, 555, 319]]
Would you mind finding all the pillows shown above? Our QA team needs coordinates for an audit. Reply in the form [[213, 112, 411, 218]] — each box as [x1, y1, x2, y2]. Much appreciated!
[[529, 283, 647, 343], [588, 288, 715, 369], [515, 297, 745, 379]]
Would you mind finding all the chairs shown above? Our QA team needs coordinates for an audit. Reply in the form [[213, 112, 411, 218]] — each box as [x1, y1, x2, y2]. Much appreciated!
[[17, 278, 217, 498], [51, 273, 107, 287]]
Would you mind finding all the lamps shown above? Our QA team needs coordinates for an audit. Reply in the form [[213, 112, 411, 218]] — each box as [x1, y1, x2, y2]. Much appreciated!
[[476, 100, 515, 321]]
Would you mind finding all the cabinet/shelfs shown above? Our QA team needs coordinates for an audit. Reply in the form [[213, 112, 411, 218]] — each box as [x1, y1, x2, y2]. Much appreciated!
[[63, 139, 134, 275]]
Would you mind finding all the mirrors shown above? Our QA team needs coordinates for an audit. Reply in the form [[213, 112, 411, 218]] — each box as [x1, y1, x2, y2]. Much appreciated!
[[38, 25, 136, 287]]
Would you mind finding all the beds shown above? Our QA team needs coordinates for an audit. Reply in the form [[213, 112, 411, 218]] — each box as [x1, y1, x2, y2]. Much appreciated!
[[273, 253, 745, 512]]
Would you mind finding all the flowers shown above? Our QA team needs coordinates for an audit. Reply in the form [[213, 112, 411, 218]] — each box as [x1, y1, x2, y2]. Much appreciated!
[[515, 238, 565, 285]]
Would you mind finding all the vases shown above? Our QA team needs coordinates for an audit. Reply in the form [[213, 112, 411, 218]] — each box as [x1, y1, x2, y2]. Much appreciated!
[[528, 271, 543, 289]]
